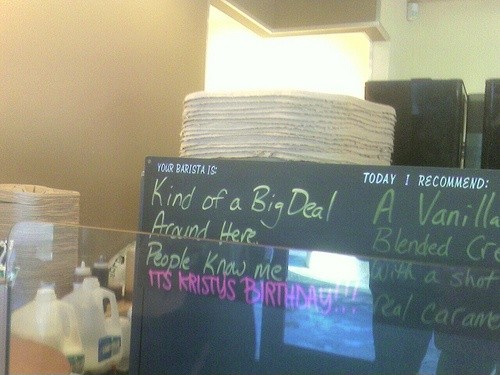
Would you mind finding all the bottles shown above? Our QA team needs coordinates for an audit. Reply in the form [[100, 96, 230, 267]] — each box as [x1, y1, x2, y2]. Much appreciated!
[[32, 254, 120, 373]]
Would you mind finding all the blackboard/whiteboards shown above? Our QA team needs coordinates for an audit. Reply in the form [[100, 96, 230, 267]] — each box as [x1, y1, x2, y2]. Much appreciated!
[[126, 155, 500, 375]]
